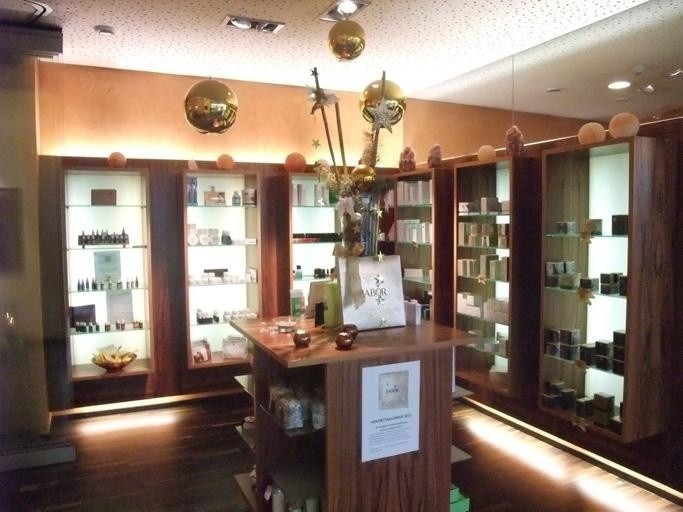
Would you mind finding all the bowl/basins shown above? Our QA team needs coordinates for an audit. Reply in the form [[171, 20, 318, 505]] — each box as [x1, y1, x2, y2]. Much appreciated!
[[272, 314, 299, 333], [91, 352, 137, 374]]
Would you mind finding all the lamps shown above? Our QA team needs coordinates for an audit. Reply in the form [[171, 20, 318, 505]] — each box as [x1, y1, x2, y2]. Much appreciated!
[[315, 1, 371, 23], [221, 15, 286, 36]]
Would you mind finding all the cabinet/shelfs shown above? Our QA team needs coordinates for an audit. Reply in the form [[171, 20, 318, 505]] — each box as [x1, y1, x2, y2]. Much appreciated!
[[63, 157, 156, 382], [393, 167, 453, 323], [183, 170, 264, 370], [277, 174, 342, 316], [228, 311, 479, 511], [453, 156, 541, 397], [539, 136, 678, 442]]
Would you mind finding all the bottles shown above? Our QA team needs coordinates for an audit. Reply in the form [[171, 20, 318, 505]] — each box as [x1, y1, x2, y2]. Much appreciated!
[[250, 462, 323, 512], [292, 265, 303, 279], [196, 307, 258, 324], [73, 226, 143, 332], [231, 191, 241, 208], [242, 189, 256, 207], [241, 416, 258, 441]]
